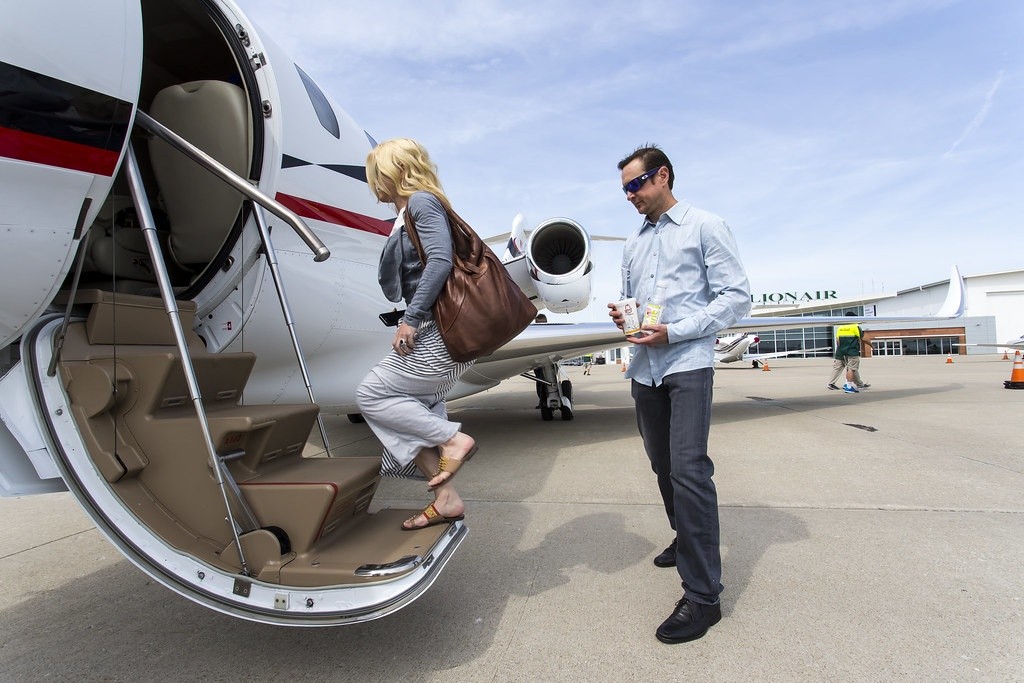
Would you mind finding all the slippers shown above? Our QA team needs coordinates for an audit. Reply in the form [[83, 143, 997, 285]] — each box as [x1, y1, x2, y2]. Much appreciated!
[[401, 501, 467, 531], [426, 442, 479, 492]]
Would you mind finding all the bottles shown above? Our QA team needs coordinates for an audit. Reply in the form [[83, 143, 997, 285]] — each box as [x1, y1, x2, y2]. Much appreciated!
[[641, 279, 669, 334]]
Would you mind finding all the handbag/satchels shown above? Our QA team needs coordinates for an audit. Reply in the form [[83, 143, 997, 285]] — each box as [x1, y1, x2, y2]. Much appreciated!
[[403, 243, 540, 363]]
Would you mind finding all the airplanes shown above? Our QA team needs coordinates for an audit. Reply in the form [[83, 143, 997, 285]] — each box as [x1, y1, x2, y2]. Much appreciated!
[[711, 333, 832, 368], [0, 0, 973, 626], [952, 334, 1024, 351]]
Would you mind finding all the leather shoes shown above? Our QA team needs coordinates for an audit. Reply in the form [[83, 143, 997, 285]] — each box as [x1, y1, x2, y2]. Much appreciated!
[[655, 596, 722, 644], [653, 535, 679, 567]]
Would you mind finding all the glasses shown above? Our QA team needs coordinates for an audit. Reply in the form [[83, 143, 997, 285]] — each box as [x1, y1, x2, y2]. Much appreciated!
[[622, 168, 658, 195]]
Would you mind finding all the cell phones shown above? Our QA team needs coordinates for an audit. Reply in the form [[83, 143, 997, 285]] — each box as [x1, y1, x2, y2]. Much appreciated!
[[378, 309, 406, 327]]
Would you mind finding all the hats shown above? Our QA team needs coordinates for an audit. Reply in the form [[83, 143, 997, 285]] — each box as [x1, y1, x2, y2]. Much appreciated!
[[845, 312, 859, 316]]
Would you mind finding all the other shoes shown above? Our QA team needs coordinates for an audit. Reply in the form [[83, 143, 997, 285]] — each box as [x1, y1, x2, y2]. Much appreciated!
[[857, 383, 872, 390]]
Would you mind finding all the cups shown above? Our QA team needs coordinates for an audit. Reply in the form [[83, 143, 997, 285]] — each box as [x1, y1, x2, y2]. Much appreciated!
[[615, 297, 640, 335]]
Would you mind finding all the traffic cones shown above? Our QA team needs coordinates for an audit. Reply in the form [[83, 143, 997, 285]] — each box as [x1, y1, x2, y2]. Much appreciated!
[[1001, 351, 1009, 360], [620, 361, 627, 372], [1002, 351, 1024, 390], [761, 358, 772, 371], [945, 352, 954, 364]]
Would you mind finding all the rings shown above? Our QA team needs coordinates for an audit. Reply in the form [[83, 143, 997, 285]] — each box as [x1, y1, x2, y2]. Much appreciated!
[[400, 339, 406, 346]]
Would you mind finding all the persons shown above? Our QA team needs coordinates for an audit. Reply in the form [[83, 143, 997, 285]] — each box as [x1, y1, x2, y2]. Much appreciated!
[[356, 137, 478, 531], [607, 143, 751, 645], [584, 353, 592, 375], [828, 312, 874, 393]]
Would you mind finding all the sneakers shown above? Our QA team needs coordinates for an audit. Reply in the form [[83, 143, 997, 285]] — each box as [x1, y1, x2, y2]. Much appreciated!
[[843, 384, 847, 389], [827, 384, 840, 390], [845, 386, 860, 394]]
